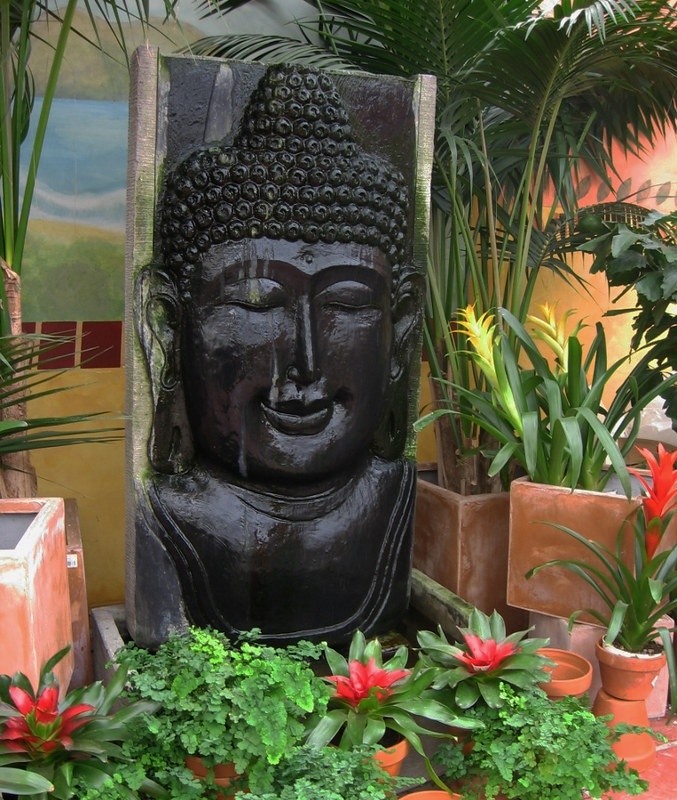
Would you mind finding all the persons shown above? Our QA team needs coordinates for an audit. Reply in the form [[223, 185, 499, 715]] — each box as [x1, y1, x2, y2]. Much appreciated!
[[136, 62, 428, 666]]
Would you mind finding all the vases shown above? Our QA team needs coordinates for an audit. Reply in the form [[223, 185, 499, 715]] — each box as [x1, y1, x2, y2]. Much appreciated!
[[367, 737, 411, 779], [590, 688, 658, 768], [397, 788, 467, 800], [591, 633, 668, 700], [505, 464, 677, 631]]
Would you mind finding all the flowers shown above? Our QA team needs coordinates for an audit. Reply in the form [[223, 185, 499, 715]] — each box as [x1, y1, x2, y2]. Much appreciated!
[[522, 441, 677, 726], [410, 297, 677, 494], [409, 607, 558, 708], [0, 643, 170, 800], [295, 629, 487, 797]]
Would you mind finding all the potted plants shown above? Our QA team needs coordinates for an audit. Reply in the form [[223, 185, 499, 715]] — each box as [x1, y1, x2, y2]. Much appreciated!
[[0, 268, 138, 706], [104, 0, 676, 800]]
